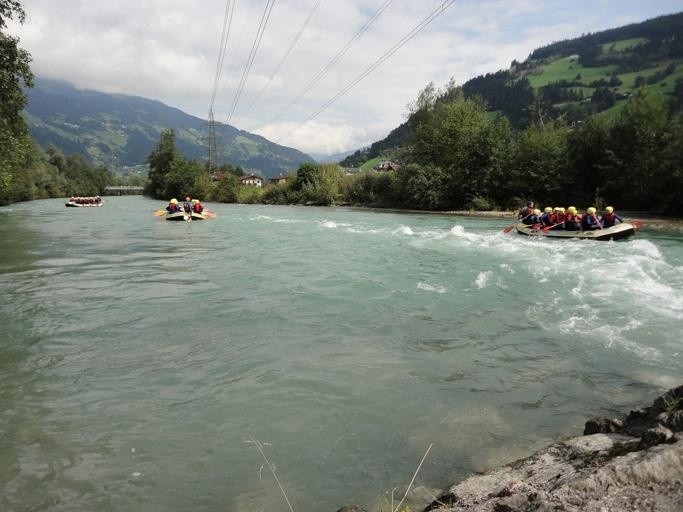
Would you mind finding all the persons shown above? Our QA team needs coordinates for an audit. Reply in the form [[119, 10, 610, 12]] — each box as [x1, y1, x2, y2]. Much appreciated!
[[191, 199, 202, 213], [182, 197, 194, 214], [165, 198, 181, 214], [69, 195, 101, 205], [517, 201, 623, 232]]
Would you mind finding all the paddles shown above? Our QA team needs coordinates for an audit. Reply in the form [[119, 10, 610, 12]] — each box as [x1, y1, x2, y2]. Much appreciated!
[[201, 208, 217, 218], [151, 209, 171, 216], [624, 220, 644, 228], [542, 221, 564, 232], [533, 223, 543, 229], [503, 213, 532, 234]]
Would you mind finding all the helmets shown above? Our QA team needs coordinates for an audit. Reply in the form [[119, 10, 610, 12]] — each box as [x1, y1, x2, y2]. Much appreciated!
[[531, 205, 614, 217], [170, 197, 199, 204]]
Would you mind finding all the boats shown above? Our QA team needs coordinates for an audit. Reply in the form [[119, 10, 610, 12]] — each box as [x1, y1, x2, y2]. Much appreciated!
[[65, 201, 105, 207], [516, 222, 637, 241], [166, 209, 208, 220]]
